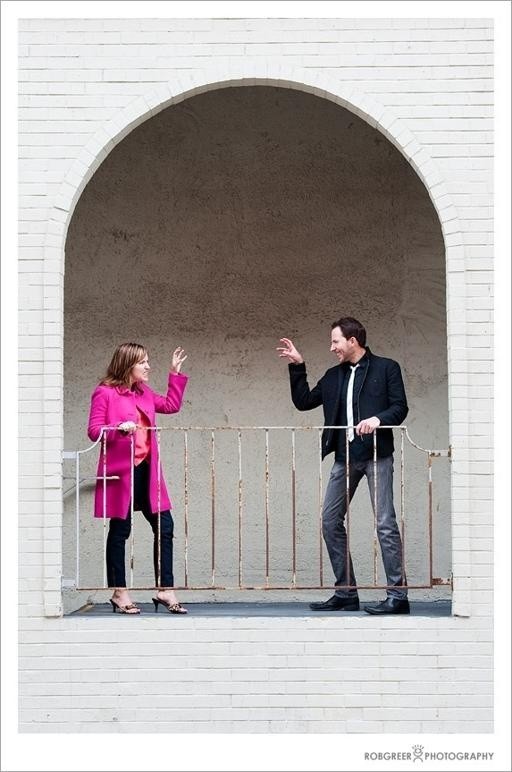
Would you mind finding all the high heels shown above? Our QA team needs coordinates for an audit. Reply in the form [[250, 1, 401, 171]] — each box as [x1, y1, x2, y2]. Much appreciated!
[[152, 598, 188, 615], [109, 598, 141, 615]]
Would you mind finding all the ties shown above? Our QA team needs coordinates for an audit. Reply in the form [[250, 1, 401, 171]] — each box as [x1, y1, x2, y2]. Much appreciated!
[[346, 363, 360, 443]]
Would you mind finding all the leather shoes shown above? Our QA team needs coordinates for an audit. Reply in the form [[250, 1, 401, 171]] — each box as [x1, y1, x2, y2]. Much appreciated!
[[309, 595, 360, 612], [363, 597, 411, 615]]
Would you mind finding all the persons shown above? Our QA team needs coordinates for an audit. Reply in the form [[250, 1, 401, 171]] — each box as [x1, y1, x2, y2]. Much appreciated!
[[276, 317, 410, 614], [87, 343, 188, 614]]
